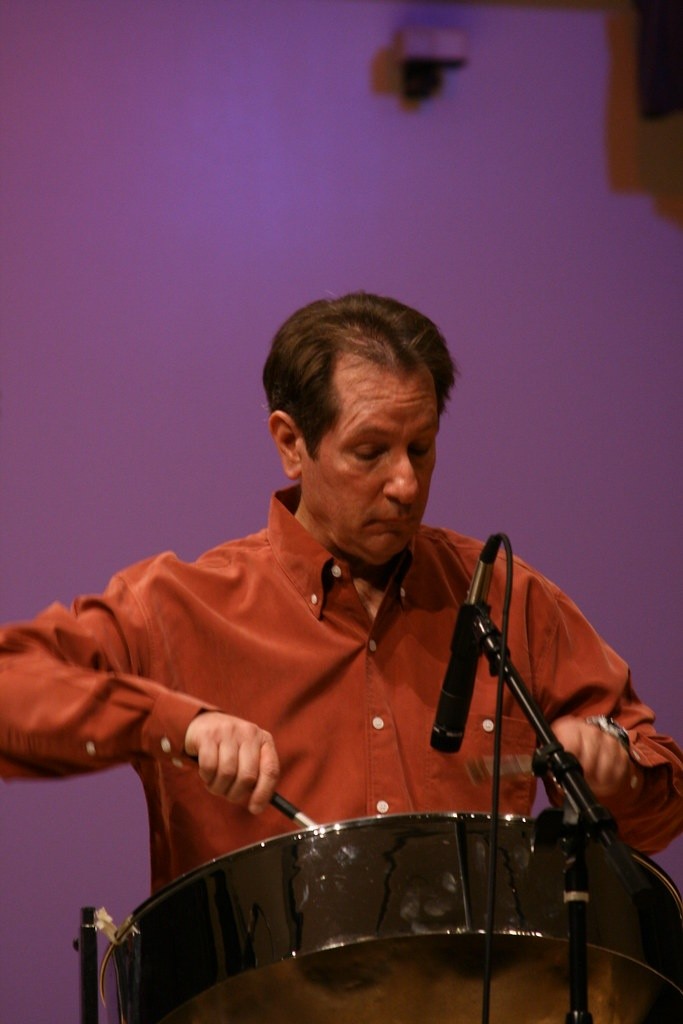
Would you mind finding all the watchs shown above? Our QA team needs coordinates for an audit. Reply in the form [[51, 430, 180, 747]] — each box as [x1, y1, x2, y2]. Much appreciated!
[[585, 713, 630, 755]]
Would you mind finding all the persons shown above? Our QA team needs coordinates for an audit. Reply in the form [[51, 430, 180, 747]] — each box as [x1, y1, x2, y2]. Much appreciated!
[[0, 293, 683, 897]]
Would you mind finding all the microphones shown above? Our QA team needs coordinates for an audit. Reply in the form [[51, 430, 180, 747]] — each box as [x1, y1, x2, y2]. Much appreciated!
[[430, 536, 501, 754]]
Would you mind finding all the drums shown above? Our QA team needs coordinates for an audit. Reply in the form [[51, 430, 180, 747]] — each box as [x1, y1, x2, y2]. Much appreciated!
[[108, 813, 683, 1024]]
